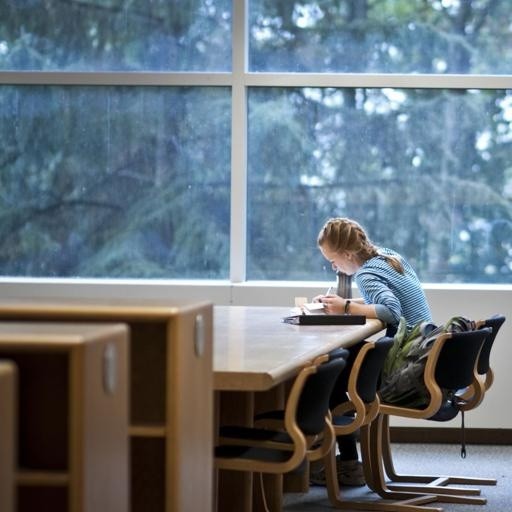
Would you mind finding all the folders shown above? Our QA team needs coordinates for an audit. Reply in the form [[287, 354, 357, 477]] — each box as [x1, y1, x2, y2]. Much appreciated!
[[283, 316, 367, 326]]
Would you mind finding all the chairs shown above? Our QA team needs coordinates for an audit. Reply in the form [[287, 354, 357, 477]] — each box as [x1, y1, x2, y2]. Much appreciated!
[[253, 350, 349, 509], [212, 356, 350, 512], [364, 324, 495, 512], [332, 336, 442, 512], [380, 313, 508, 497]]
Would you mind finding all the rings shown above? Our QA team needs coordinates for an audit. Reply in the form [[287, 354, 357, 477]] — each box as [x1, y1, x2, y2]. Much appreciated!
[[323, 304, 330, 309]]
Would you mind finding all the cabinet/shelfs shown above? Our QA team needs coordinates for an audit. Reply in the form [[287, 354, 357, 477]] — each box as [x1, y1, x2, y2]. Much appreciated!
[[0, 298, 209, 510]]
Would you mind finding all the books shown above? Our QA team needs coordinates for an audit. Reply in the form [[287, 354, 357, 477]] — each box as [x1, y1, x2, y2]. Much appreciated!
[[303, 302, 329, 315], [282, 313, 366, 326]]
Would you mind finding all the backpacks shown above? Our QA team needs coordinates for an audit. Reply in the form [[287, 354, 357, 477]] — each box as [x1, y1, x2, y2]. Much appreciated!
[[379, 315, 475, 404]]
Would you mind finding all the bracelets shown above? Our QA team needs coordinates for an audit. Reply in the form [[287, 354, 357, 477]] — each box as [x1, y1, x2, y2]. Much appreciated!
[[345, 297, 351, 315]]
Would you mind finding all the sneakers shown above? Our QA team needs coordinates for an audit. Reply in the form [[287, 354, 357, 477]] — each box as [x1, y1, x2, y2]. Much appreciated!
[[309, 459, 367, 487]]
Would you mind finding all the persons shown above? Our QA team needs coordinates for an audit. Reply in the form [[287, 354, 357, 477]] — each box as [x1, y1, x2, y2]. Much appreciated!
[[308, 215, 436, 488]]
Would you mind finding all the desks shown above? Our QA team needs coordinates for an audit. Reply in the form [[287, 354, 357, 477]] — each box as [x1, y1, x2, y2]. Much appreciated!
[[213, 305, 387, 512]]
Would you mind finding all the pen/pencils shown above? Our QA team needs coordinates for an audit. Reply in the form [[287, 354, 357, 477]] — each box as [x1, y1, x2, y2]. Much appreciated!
[[326, 286, 333, 296]]
